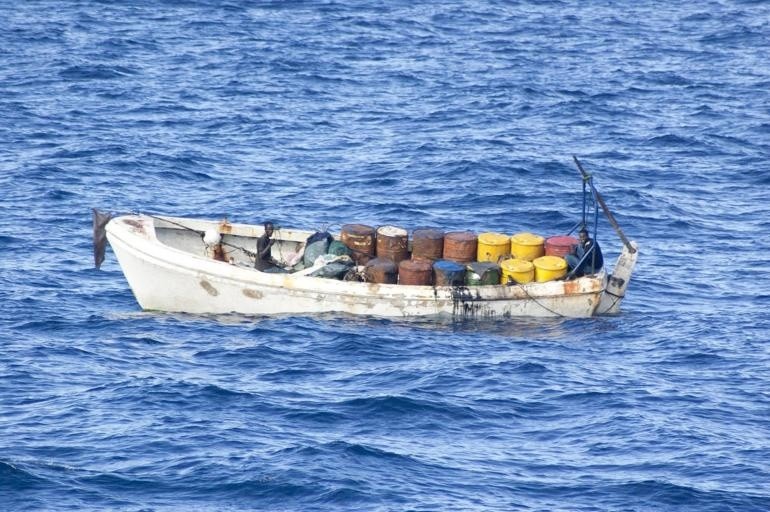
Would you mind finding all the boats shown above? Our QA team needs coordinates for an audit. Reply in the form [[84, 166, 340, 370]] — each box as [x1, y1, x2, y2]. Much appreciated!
[[104, 155, 641, 321]]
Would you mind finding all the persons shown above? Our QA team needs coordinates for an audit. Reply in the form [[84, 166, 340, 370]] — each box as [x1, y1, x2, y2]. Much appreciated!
[[254, 221, 298, 273], [564, 228, 603, 276]]
